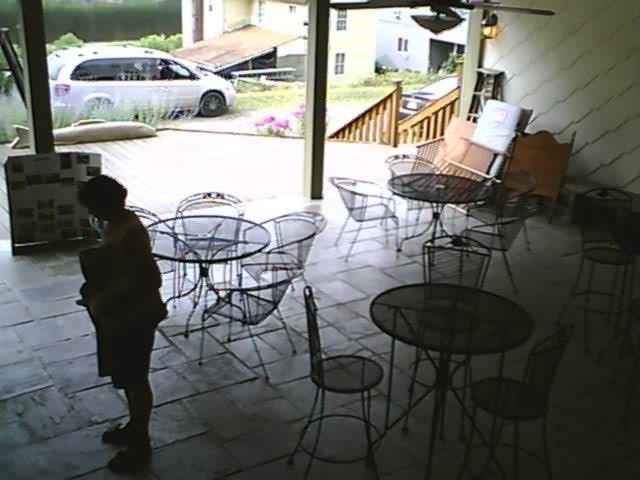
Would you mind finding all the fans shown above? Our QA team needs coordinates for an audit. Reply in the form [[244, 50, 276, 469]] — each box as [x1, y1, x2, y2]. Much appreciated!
[[326, 0, 557, 19]]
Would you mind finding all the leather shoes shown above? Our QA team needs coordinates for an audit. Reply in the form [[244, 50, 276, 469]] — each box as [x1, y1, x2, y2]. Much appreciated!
[[101, 423, 140, 446], [109, 448, 153, 472]]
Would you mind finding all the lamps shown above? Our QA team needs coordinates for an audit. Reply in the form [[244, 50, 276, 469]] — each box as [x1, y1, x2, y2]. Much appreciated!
[[410, 12, 465, 35], [480, 13, 499, 40]]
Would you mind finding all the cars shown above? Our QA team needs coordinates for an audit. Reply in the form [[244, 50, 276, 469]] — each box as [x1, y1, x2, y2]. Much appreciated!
[[399, 75, 459, 121], [46, 46, 238, 120]]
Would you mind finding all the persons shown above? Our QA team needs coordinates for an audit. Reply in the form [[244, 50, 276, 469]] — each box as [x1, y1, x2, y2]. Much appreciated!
[[73, 173, 169, 472]]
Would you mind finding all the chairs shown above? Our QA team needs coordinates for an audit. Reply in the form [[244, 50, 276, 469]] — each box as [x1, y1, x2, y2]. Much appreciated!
[[498, 126, 576, 225]]
